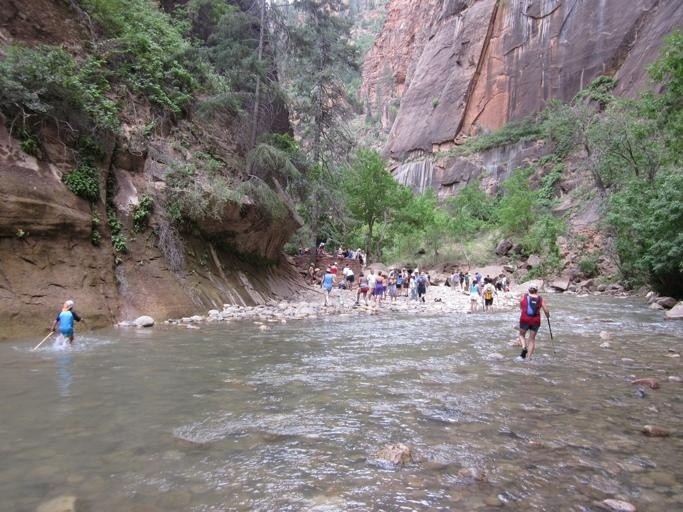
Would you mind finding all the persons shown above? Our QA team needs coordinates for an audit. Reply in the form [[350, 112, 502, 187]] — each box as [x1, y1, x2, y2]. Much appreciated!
[[518, 283, 550, 359], [51, 300, 81, 345], [309, 242, 509, 313]]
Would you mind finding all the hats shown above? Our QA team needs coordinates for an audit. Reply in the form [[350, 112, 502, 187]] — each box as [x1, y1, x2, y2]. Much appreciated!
[[530, 283, 538, 290]]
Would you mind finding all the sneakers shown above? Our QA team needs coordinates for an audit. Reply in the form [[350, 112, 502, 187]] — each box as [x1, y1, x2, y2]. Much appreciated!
[[521, 347, 527, 359]]
